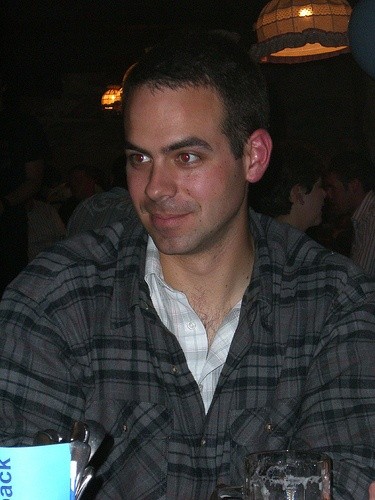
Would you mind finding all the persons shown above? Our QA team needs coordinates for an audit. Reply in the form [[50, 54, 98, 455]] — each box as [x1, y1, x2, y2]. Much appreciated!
[[248, 130, 327, 232], [324, 151, 375, 274], [0, 33, 375, 500], [27, 165, 137, 262], [0, 65, 49, 299]]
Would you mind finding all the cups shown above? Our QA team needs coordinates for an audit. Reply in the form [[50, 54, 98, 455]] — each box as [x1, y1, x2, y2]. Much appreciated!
[[243, 448, 332, 500]]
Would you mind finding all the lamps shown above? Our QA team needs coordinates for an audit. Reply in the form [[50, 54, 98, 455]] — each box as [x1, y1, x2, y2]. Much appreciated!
[[100, 83, 126, 111], [255, 1, 352, 64]]
